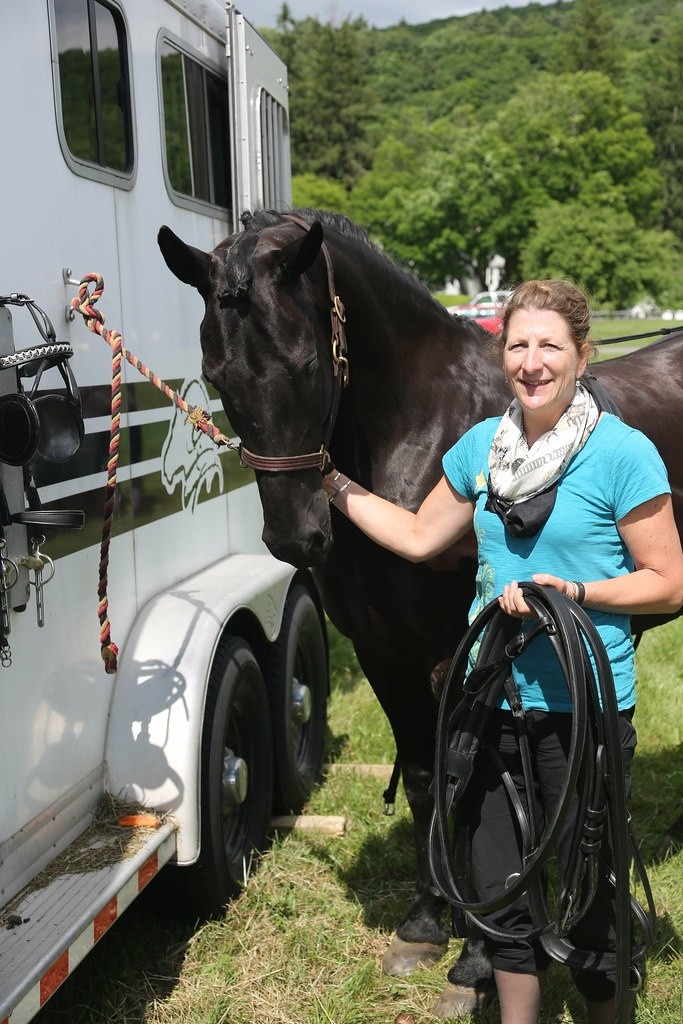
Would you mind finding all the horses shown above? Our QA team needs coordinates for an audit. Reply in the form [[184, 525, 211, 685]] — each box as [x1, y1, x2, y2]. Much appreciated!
[[156, 210, 683, 1023]]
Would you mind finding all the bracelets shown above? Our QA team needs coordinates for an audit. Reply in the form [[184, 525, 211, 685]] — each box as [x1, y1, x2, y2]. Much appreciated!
[[569, 580, 576, 600], [325, 473, 340, 495], [330, 480, 351, 501], [574, 582, 585, 606]]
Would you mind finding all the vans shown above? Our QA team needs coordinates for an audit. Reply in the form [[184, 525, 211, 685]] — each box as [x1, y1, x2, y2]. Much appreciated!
[[0, 0, 335, 1023]]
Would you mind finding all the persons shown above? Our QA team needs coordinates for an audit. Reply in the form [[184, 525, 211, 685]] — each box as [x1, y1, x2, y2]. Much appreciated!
[[322, 279, 683, 1024]]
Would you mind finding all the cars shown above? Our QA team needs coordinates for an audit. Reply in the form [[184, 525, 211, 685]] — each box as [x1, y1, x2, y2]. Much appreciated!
[[442, 289, 515, 338]]
